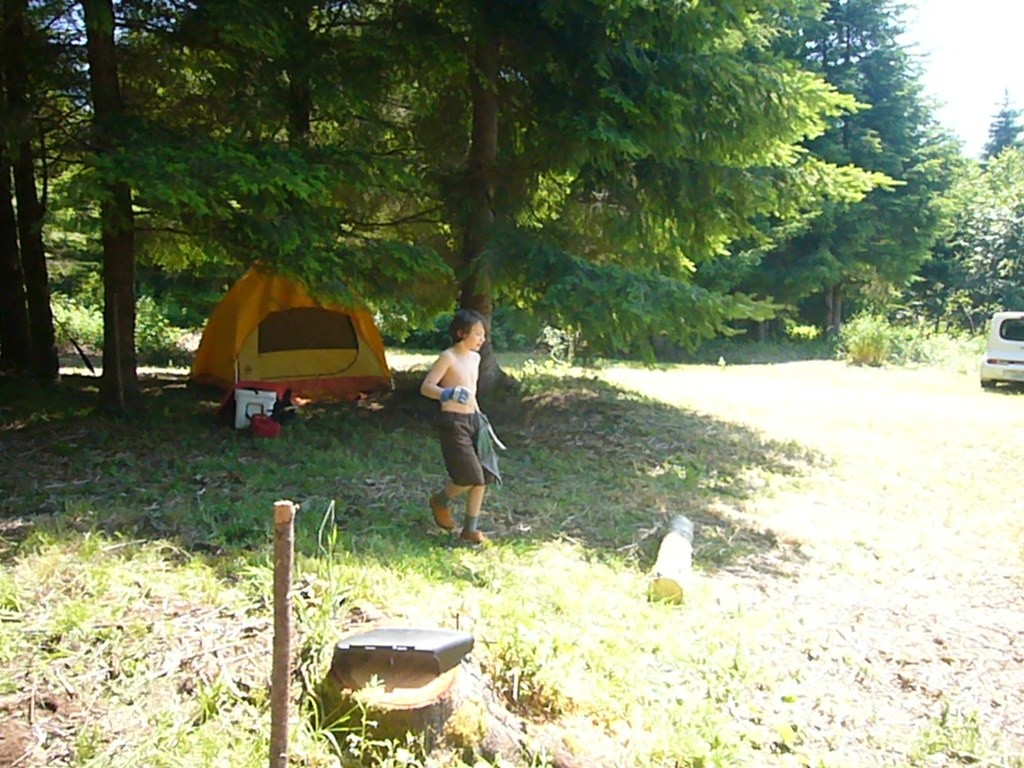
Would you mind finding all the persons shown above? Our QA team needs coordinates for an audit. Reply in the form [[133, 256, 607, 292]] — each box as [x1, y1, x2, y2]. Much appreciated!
[[421, 307, 486, 548]]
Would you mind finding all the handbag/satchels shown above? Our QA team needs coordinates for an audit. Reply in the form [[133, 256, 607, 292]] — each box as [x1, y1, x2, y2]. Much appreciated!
[[250, 414, 280, 438], [270, 400, 294, 421]]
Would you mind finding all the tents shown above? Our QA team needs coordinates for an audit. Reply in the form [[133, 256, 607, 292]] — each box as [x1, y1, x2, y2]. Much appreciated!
[[191, 261, 389, 408]]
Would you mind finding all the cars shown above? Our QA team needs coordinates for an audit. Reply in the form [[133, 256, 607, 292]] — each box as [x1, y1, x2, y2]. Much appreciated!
[[979, 311, 1024, 388]]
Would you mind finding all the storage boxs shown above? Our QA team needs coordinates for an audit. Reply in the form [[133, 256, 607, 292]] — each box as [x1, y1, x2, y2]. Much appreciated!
[[234, 389, 277, 429]]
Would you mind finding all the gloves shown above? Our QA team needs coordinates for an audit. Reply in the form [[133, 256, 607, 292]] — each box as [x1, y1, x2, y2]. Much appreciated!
[[441, 386, 471, 404]]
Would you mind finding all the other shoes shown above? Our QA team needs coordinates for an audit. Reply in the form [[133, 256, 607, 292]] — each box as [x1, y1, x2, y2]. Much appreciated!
[[460, 530, 484, 542], [429, 495, 455, 529]]
